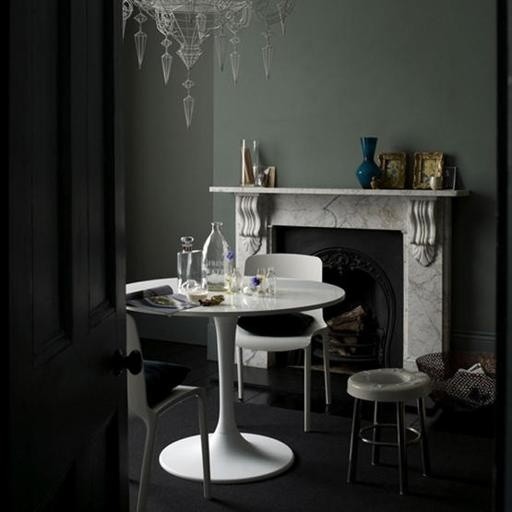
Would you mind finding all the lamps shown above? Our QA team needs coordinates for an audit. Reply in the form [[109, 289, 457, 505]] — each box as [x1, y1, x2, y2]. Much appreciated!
[[121, 0, 299, 132]]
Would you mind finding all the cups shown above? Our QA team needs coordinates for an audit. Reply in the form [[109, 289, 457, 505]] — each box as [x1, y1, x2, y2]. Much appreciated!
[[252, 164, 271, 188], [181, 278, 208, 306], [222, 248, 245, 294], [430, 176, 441, 190]]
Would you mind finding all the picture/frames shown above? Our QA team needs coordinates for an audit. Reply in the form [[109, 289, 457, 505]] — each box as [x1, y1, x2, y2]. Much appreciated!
[[413, 152, 444, 190], [377, 151, 407, 188]]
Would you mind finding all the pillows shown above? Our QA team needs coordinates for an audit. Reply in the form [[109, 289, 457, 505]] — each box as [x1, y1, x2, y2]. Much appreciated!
[[237, 312, 314, 337], [143, 366, 191, 408]]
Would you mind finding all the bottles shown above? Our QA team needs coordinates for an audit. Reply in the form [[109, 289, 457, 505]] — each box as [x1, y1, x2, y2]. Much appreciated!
[[201, 222, 232, 292], [240, 138, 259, 187], [176, 236, 208, 300], [231, 265, 277, 297]]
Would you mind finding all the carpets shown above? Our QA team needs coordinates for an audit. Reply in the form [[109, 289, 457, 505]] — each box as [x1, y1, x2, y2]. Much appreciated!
[[128, 394, 510, 511]]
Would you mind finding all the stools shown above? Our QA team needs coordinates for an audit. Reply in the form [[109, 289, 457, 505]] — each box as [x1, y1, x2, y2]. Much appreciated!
[[346, 367, 432, 496]]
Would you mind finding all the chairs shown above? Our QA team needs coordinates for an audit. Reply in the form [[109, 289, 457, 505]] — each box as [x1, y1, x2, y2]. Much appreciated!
[[125, 313, 213, 512], [234, 252, 332, 434]]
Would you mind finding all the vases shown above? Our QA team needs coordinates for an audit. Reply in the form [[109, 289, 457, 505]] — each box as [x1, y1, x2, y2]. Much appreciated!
[[356, 136, 379, 188]]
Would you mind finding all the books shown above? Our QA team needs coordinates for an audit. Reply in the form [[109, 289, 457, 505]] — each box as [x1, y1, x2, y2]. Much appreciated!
[[124, 283, 201, 317]]
